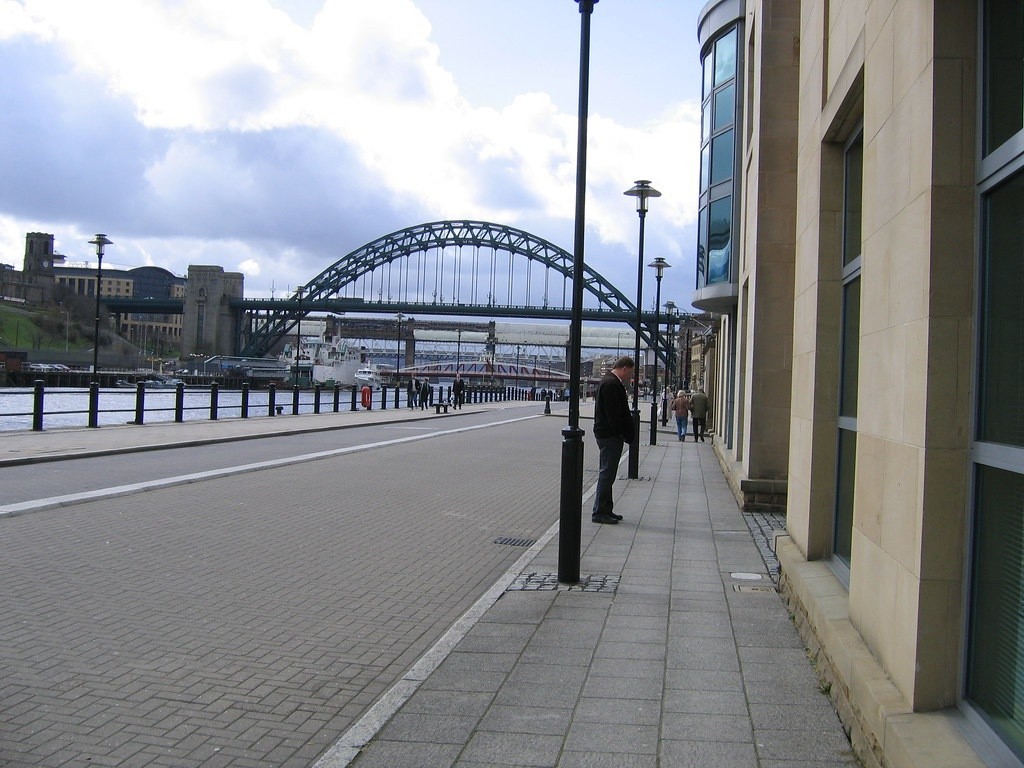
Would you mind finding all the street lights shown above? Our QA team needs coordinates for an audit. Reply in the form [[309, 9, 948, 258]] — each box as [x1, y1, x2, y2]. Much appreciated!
[[558, 1, 600, 584], [88, 234, 113, 428], [456, 327, 465, 374], [623, 179, 661, 481], [659, 301, 676, 424], [646, 256, 672, 449], [292, 284, 307, 416], [395, 311, 405, 409]]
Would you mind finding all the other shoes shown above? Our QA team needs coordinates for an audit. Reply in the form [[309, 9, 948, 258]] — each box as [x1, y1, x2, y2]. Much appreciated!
[[701, 437, 705, 442], [678, 435, 685, 441], [695, 439, 698, 442]]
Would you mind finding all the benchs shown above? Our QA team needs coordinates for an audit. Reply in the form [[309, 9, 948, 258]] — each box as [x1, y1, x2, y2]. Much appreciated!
[[429, 403, 452, 413]]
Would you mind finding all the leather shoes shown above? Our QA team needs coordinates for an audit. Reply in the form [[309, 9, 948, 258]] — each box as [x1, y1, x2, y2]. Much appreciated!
[[592, 517, 618, 523], [605, 513, 623, 519]]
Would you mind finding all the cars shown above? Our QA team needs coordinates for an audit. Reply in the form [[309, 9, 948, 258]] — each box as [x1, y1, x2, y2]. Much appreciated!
[[27, 361, 71, 372]]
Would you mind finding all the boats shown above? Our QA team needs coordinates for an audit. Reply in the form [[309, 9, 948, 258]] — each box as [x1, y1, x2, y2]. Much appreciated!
[[115, 317, 403, 389]]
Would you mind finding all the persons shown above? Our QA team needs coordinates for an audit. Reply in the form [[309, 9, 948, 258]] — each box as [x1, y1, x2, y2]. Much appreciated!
[[451, 372, 464, 411], [528, 385, 567, 402], [673, 391, 689, 441], [591, 356, 635, 524], [407, 373, 418, 411], [420, 378, 430, 412], [657, 386, 674, 424], [689, 386, 709, 442], [591, 388, 597, 400]]
